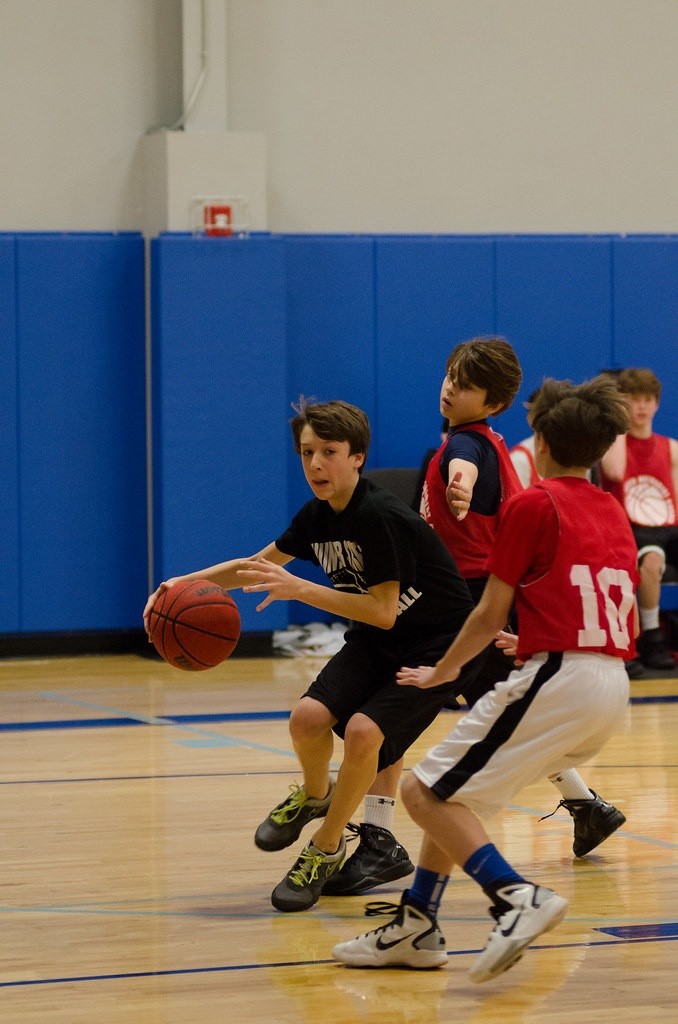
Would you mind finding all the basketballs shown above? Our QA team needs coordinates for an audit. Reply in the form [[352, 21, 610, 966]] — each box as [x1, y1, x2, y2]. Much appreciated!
[[149, 579, 242, 671]]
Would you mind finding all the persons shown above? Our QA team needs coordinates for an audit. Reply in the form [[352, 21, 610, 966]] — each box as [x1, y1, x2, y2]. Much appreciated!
[[328, 374, 642, 983], [319, 339, 626, 895], [142, 393, 471, 911], [508, 370, 678, 676]]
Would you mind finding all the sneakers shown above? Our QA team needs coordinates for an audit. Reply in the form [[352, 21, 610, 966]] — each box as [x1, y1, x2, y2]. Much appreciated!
[[332, 887, 449, 969], [469, 884, 568, 982], [255, 775, 339, 851], [539, 787, 627, 859], [321, 821, 415, 897], [270, 829, 346, 912]]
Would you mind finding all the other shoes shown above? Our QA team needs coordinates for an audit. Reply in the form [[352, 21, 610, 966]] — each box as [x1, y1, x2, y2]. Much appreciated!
[[624, 659, 645, 682], [637, 628, 676, 668]]
